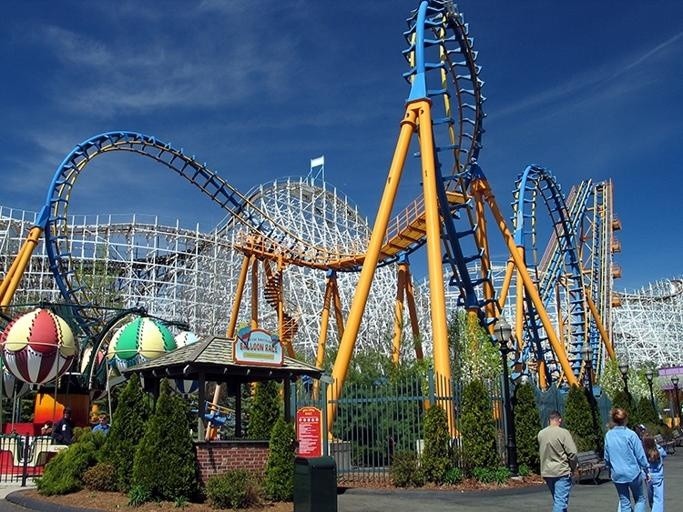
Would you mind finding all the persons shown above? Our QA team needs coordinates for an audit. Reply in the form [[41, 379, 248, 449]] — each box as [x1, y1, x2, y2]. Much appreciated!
[[52, 407, 74, 444], [607, 420, 622, 512], [537, 409, 579, 512], [639, 432, 668, 512], [602, 406, 651, 512], [91, 411, 110, 434], [40, 422, 52, 435]]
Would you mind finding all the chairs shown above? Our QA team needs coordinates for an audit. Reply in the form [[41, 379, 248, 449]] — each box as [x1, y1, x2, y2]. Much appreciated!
[[0, 435, 69, 481]]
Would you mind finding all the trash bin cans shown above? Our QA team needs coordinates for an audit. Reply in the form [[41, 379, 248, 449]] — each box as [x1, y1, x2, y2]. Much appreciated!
[[293, 455, 338, 512]]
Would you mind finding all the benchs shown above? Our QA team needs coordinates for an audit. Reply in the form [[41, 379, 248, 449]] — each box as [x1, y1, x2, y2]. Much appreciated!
[[576, 450, 611, 485], [672, 430, 683, 446], [654, 434, 676, 453]]
